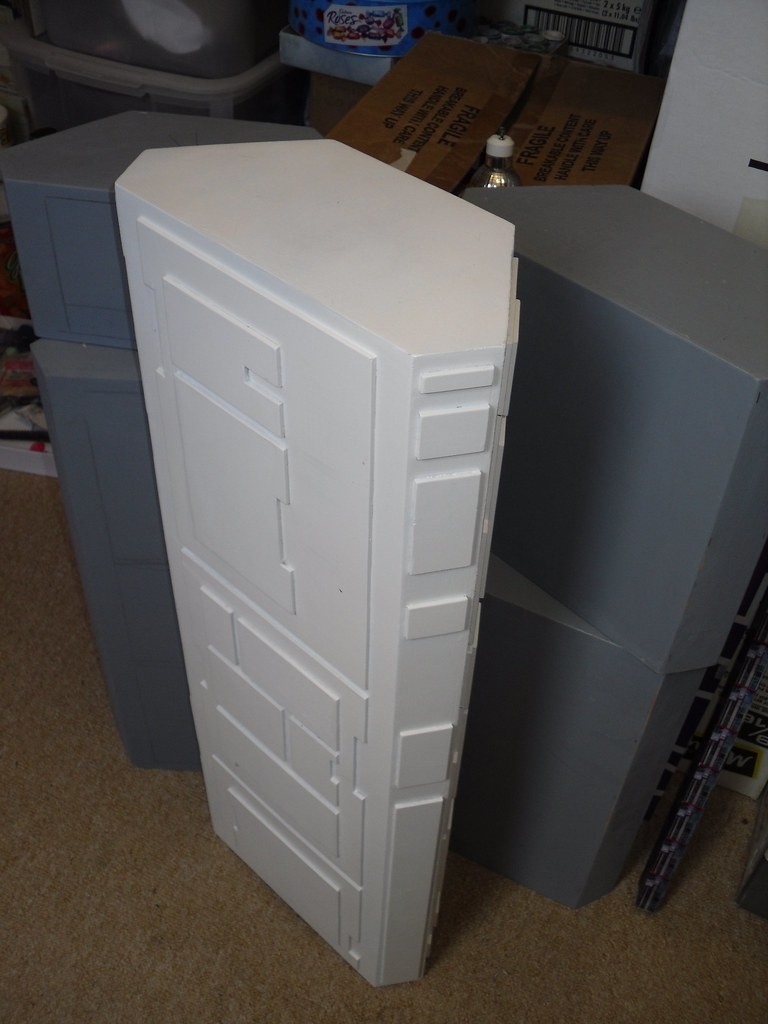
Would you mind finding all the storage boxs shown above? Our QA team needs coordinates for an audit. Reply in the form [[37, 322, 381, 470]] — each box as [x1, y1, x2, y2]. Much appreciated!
[[0, 0, 768, 251], [643, 539, 768, 918]]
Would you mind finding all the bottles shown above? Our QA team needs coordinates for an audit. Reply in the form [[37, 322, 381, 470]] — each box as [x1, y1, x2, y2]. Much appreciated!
[[470, 127, 523, 189]]
